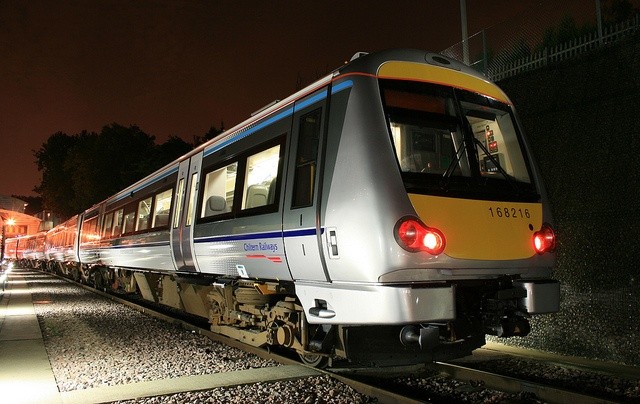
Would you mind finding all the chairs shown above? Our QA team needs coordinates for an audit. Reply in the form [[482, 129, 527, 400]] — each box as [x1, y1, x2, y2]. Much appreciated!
[[155, 213, 170, 228], [138, 214, 151, 230], [104, 227, 111, 236], [124, 220, 136, 232], [114, 227, 123, 236], [204, 195, 228, 217], [242, 184, 270, 209]]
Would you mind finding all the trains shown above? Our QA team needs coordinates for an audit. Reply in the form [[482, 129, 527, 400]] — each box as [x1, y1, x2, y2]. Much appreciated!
[[0, 45, 562, 368]]
